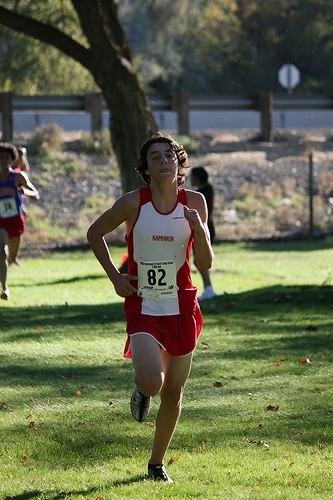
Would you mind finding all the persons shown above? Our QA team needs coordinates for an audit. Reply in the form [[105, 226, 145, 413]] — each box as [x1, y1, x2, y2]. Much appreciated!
[[87, 136, 215, 487], [190, 166, 215, 300], [0, 140, 40, 300]]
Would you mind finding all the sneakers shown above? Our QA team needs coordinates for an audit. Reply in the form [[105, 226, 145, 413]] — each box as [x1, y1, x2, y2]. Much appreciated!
[[147, 461, 173, 483], [130, 386, 151, 422], [0, 290, 8, 300]]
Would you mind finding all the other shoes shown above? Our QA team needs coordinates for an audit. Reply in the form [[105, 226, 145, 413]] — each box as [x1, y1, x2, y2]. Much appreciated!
[[198, 291, 216, 300]]
